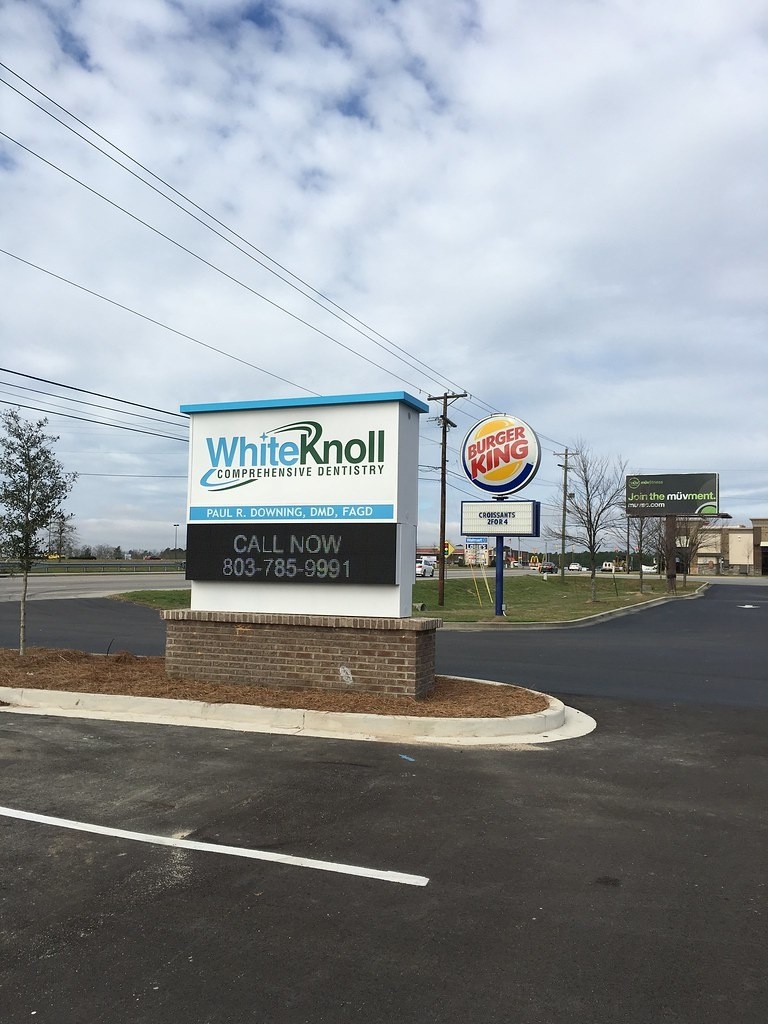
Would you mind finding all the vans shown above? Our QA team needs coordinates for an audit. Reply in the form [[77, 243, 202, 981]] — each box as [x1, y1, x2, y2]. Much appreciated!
[[602, 561, 614, 572]]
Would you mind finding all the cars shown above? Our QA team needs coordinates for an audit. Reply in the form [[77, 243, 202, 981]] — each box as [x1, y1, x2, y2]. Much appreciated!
[[539, 561, 559, 574], [176, 560, 186, 571], [47, 552, 65, 559], [569, 562, 583, 572], [144, 554, 162, 560], [415, 558, 435, 577]]
